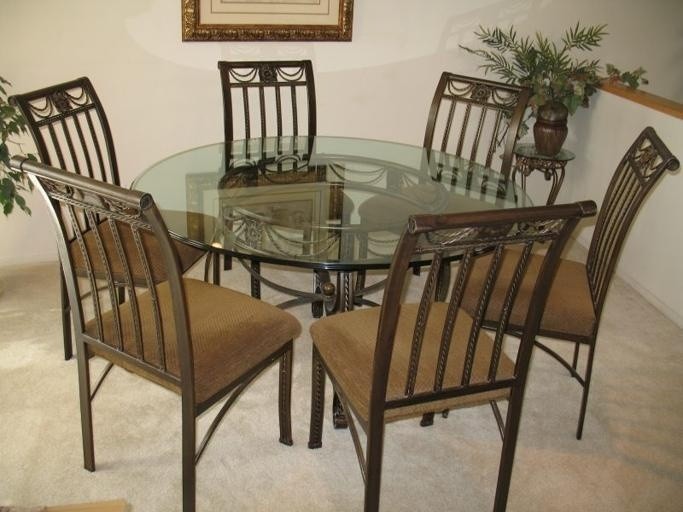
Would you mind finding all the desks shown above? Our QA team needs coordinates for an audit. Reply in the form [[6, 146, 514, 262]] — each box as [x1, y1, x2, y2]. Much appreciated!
[[127, 134, 535, 429], [511, 143, 574, 206]]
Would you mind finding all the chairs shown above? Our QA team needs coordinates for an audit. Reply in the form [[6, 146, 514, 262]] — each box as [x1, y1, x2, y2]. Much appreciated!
[[356, 72, 531, 304], [309, 201, 597, 512], [9, 157, 299, 511], [11, 77, 223, 360], [217, 59, 352, 317], [441, 125, 679, 441]]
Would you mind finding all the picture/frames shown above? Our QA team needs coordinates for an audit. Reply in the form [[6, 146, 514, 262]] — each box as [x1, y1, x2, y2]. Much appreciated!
[[181, 0, 354, 41]]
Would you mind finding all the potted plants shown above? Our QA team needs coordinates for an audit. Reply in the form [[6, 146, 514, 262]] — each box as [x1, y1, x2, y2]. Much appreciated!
[[458, 23, 650, 156]]
